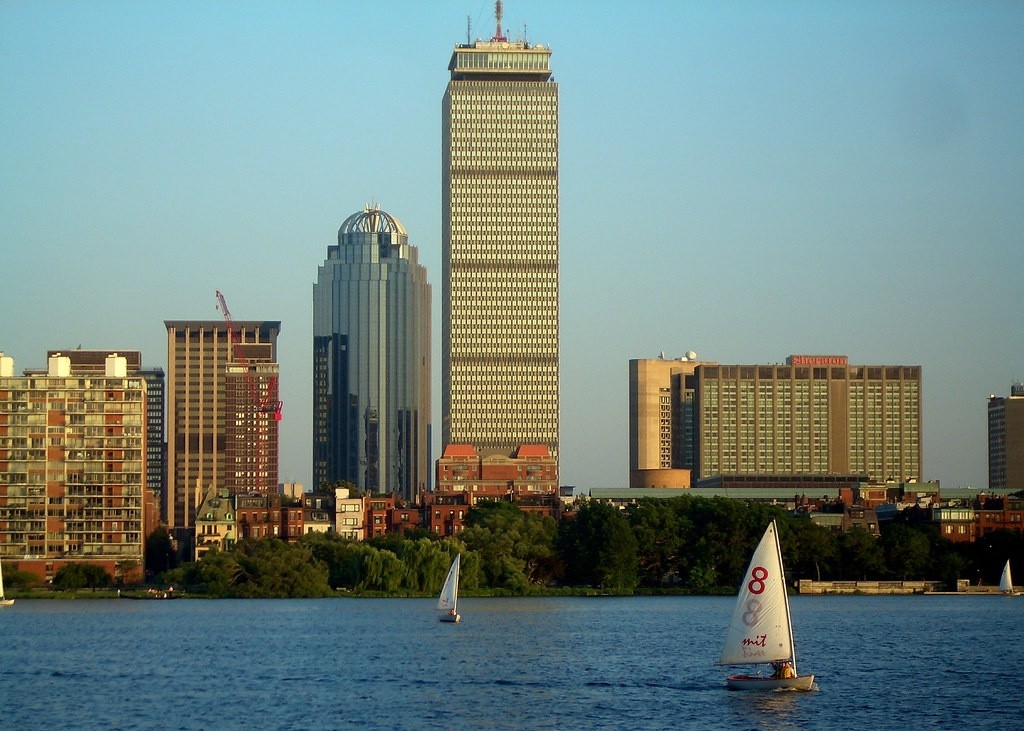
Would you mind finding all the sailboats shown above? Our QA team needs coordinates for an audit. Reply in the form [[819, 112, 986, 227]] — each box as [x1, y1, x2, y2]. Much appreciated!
[[713, 517, 815, 692], [999, 558, 1021, 596], [433, 552, 462, 624], [0, 555, 15, 607]]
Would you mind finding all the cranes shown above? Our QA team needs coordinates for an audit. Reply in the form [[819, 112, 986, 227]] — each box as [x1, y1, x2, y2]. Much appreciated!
[[214, 291, 283, 498]]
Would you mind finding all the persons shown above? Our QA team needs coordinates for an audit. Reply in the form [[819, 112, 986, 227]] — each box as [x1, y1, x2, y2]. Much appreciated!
[[770, 661, 799, 679]]
